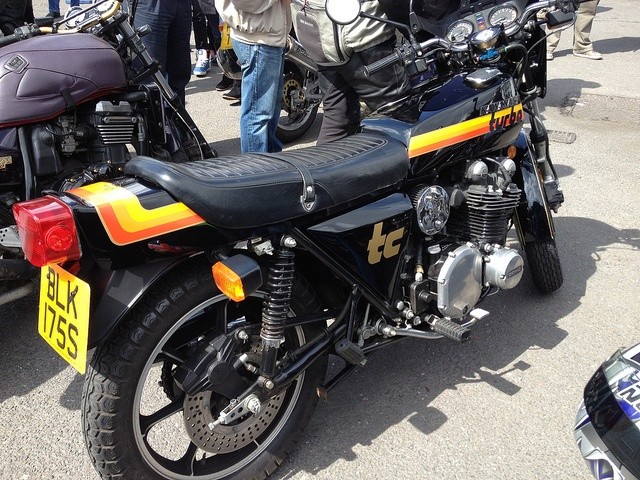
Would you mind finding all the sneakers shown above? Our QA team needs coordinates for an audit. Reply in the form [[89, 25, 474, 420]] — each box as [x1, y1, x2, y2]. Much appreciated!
[[45, 12, 60, 19], [216, 75, 233, 90], [573, 51, 602, 60], [212, 55, 218, 66], [193, 49, 211, 76], [546, 51, 554, 60], [222, 82, 241, 99]]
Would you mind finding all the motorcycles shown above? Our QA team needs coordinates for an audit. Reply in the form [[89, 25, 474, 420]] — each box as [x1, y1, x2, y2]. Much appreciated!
[[0, 0, 218, 306], [218, 21, 323, 144], [12, 0, 613, 480]]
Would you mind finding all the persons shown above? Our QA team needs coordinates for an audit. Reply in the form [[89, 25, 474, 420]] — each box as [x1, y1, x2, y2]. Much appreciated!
[[214, 0, 292, 155], [291, 0, 418, 147], [545, 1, 603, 61], [1, 0, 35, 36], [216, 49, 241, 100], [46, 0, 84, 19], [192, 0, 222, 77], [129, 0, 222, 113]]
